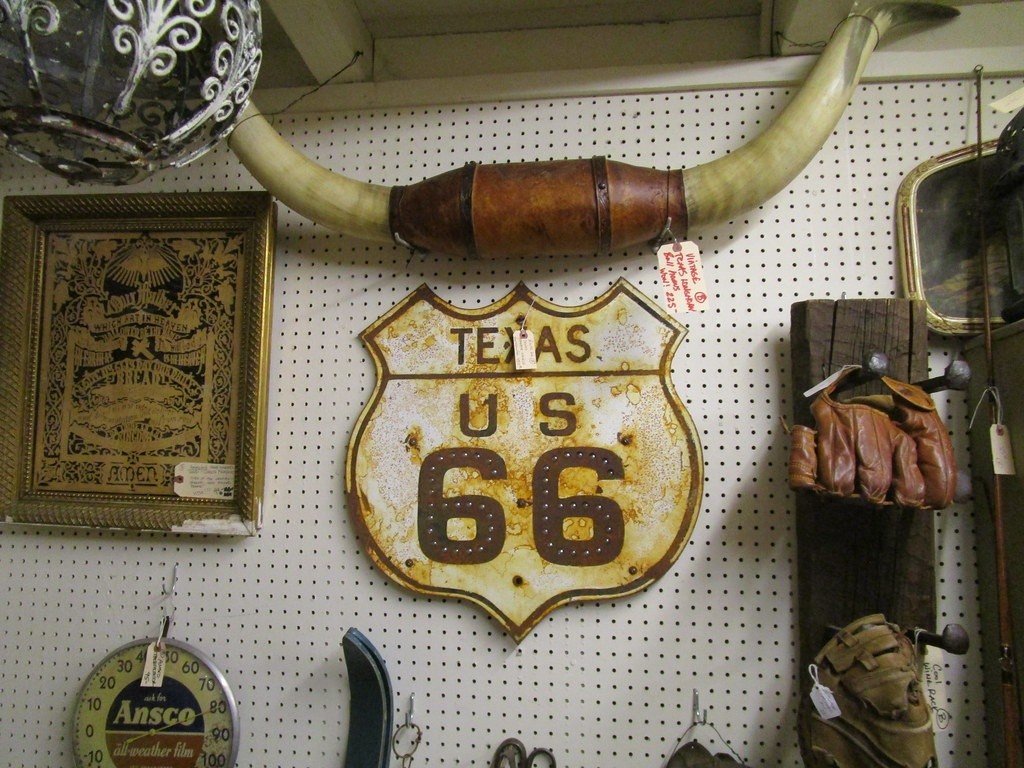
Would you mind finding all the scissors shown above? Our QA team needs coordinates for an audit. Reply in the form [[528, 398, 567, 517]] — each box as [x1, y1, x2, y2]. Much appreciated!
[[491, 738, 556, 767]]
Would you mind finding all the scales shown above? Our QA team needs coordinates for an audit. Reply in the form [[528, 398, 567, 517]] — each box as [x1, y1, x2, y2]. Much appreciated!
[[72, 636, 241, 768]]
